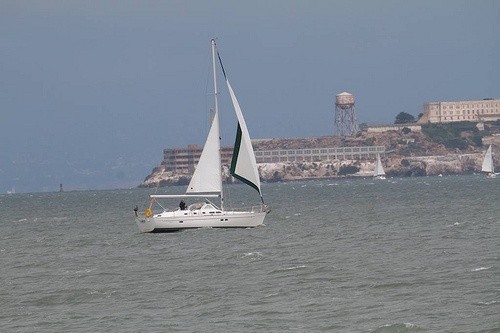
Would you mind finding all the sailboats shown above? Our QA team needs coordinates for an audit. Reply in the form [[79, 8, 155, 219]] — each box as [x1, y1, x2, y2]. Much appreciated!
[[134, 38, 270, 232], [480, 145, 499, 178], [374, 149, 387, 181]]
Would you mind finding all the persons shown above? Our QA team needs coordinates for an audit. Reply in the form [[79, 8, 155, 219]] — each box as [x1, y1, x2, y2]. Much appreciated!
[[179, 200, 187, 210]]
